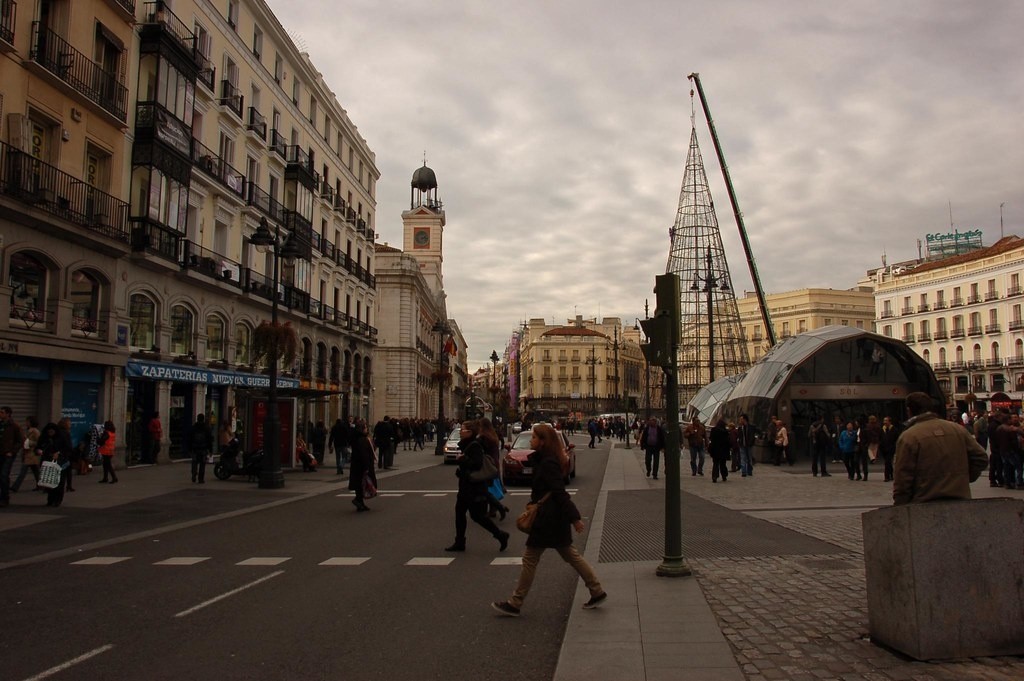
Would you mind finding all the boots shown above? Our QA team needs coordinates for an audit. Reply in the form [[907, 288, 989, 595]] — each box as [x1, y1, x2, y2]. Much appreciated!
[[862, 472, 868, 481], [856, 472, 862, 480]]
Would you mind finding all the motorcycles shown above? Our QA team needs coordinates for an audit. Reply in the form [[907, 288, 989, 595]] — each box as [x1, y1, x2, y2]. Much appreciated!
[[213, 435, 264, 480]]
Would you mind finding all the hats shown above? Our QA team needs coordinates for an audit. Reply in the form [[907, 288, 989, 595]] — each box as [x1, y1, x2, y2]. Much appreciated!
[[770, 416, 776, 419], [717, 419, 727, 425]]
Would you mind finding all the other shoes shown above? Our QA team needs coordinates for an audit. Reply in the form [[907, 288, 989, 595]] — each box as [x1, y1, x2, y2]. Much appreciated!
[[822, 471, 831, 476], [990, 480, 1024, 489], [444, 537, 465, 552], [486, 505, 510, 521], [109, 477, 119, 484], [813, 472, 817, 476], [494, 528, 510, 550], [582, 592, 606, 609], [99, 478, 108, 483], [490, 601, 520, 616], [352, 494, 370, 512]]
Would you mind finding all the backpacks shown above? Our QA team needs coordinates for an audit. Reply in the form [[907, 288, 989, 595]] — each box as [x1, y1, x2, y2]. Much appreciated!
[[815, 424, 829, 447], [307, 453, 318, 466]]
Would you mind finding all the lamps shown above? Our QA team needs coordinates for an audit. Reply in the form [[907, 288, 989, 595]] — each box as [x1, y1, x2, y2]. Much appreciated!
[[47, 196, 300, 311], [307, 308, 386, 345], [310, 176, 325, 186], [133, 11, 169, 26], [347, 214, 389, 248], [335, 202, 350, 209], [268, 138, 289, 148], [215, 90, 241, 101], [198, 61, 216, 73], [319, 189, 336, 196], [286, 158, 306, 165], [244, 116, 268, 126], [136, 345, 297, 375]]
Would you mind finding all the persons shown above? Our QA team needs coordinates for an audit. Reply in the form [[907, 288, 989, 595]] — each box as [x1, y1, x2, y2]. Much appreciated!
[[0, 404, 1024, 523], [892, 392, 989, 507], [444, 420, 510, 552], [490, 424, 608, 618]]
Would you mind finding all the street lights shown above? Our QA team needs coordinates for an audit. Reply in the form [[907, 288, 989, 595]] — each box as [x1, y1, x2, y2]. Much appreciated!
[[604, 323, 626, 413], [585, 343, 602, 419], [633, 300, 650, 422], [490, 352, 500, 433], [249, 213, 310, 493], [434, 316, 449, 456], [691, 245, 730, 384], [503, 364, 509, 428]]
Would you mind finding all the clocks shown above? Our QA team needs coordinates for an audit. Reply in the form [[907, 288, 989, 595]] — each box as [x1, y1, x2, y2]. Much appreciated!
[[414, 227, 431, 250]]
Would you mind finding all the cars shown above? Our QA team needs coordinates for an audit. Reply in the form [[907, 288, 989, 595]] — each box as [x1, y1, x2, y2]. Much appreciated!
[[444, 428, 466, 463], [501, 430, 576, 485]]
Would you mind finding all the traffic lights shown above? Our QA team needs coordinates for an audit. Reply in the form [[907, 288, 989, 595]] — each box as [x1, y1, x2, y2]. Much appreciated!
[[639, 318, 672, 367]]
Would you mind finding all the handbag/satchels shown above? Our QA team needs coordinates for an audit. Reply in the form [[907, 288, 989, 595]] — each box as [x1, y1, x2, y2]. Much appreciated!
[[362, 469, 377, 499], [22, 445, 41, 465], [462, 440, 498, 483], [517, 492, 551, 535], [37, 460, 62, 489]]
